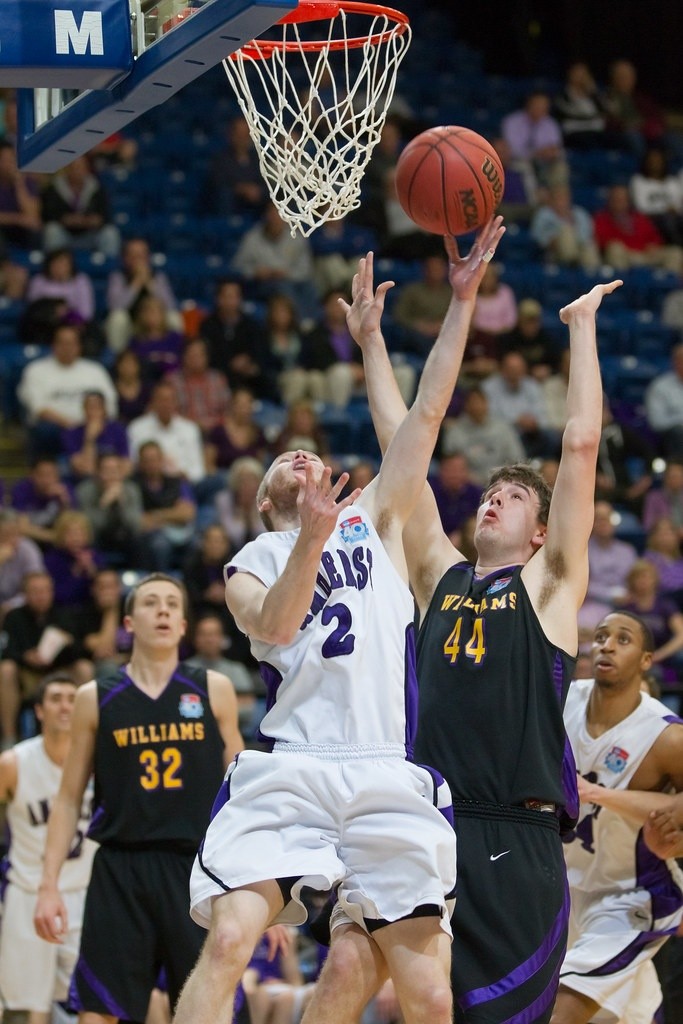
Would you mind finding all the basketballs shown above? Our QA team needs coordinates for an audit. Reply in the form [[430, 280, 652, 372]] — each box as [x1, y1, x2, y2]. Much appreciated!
[[394, 126, 507, 236]]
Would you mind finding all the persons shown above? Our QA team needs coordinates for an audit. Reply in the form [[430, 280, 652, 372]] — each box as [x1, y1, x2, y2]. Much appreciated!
[[0, 50, 683, 1024], [337, 251, 625, 1024], [170, 213, 505, 1024]]
